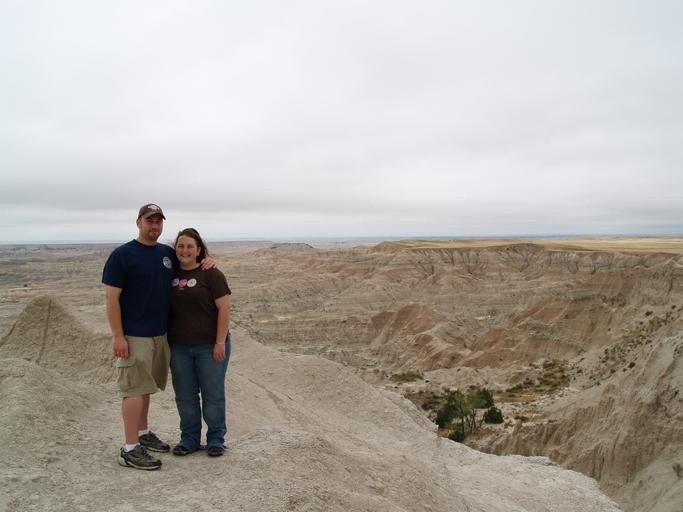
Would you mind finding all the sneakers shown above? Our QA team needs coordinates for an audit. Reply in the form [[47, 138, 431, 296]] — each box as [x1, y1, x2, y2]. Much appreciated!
[[118, 445, 162, 470], [173, 442, 199, 455], [208, 447, 224, 456], [138, 430, 170, 453]]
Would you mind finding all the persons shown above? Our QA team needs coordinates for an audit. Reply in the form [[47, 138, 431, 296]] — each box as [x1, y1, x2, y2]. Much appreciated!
[[164, 227, 231, 457], [102, 205, 216, 472]]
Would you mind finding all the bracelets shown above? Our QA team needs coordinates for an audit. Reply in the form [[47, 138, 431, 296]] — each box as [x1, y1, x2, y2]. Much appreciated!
[[214, 342, 224, 345]]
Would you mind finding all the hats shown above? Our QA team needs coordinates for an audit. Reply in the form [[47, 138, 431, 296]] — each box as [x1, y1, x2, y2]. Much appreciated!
[[139, 204, 166, 220]]
